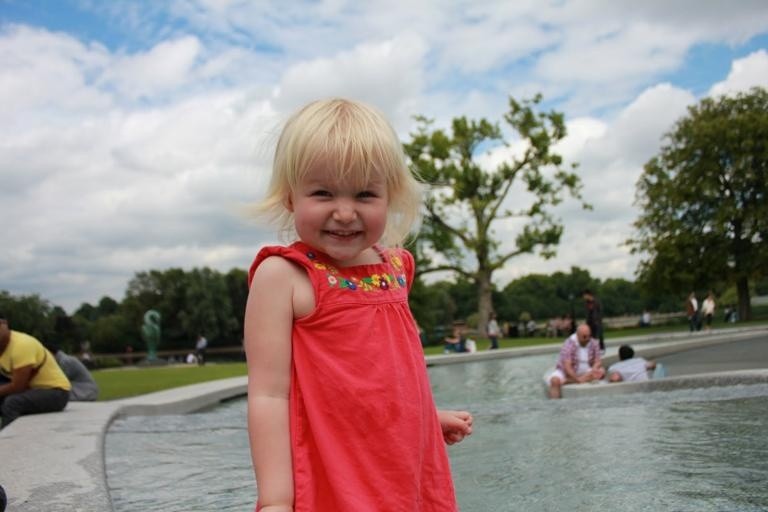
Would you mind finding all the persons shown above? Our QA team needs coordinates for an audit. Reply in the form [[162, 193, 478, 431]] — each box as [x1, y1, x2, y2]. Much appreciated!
[[0, 310, 72, 431], [53, 346, 98, 401], [78, 336, 91, 369], [126, 345, 133, 364], [167, 335, 208, 366], [241, 98, 474, 512]]
